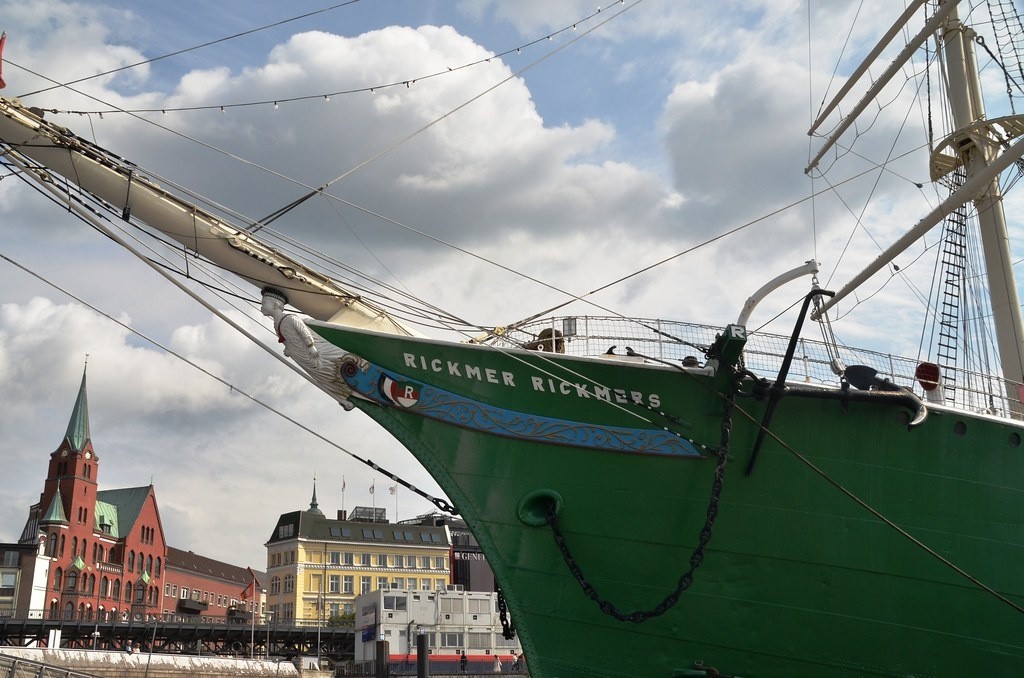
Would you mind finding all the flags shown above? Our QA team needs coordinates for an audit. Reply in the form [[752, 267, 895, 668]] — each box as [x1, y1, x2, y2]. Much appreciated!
[[389, 485, 398, 495], [241, 582, 254, 601], [369, 485, 374, 494]]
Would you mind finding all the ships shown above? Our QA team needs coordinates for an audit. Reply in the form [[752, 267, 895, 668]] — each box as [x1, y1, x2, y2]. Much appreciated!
[[2, 0, 1024, 678]]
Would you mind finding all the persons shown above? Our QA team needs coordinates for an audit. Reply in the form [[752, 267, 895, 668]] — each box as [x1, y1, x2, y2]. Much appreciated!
[[493, 654, 502, 672], [460, 650, 468, 673], [260, 286, 368, 395], [511, 652, 524, 673], [124, 639, 140, 655]]
